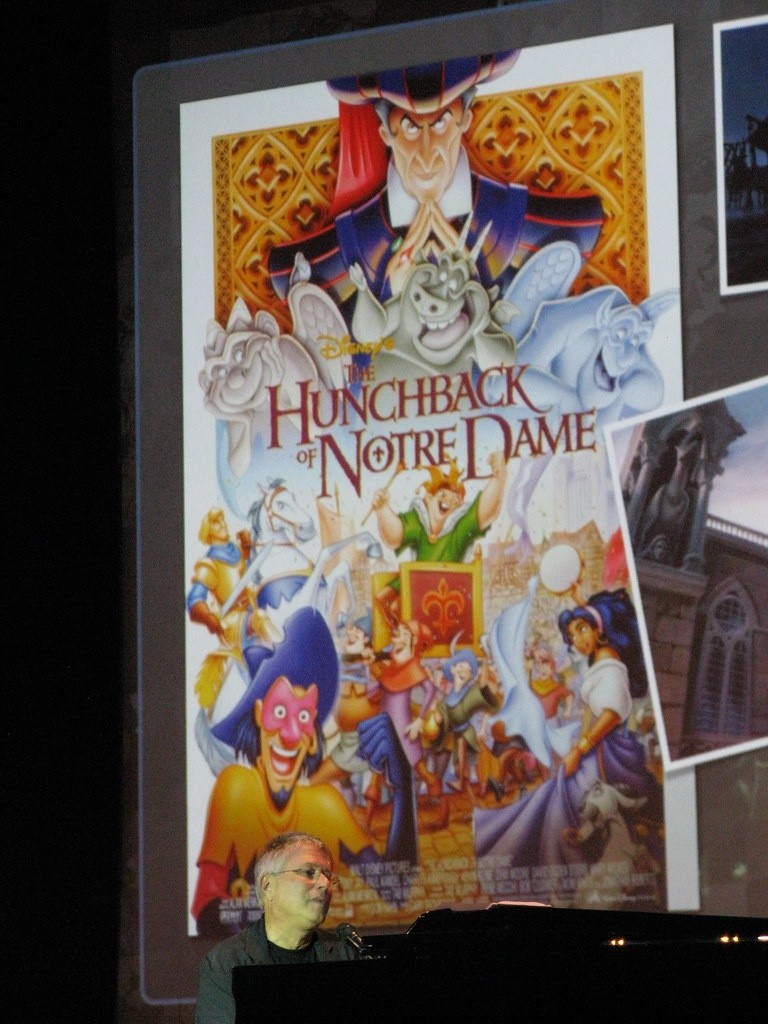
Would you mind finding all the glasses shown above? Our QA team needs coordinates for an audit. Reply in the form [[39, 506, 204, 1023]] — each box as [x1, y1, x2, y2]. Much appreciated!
[[266, 869, 340, 885]]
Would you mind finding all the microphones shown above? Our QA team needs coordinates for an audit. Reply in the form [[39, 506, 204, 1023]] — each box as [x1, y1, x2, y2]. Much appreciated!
[[335, 922, 373, 959]]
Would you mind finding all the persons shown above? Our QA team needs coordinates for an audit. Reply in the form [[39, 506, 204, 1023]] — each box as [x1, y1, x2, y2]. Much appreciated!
[[191, 834, 359, 1024]]
